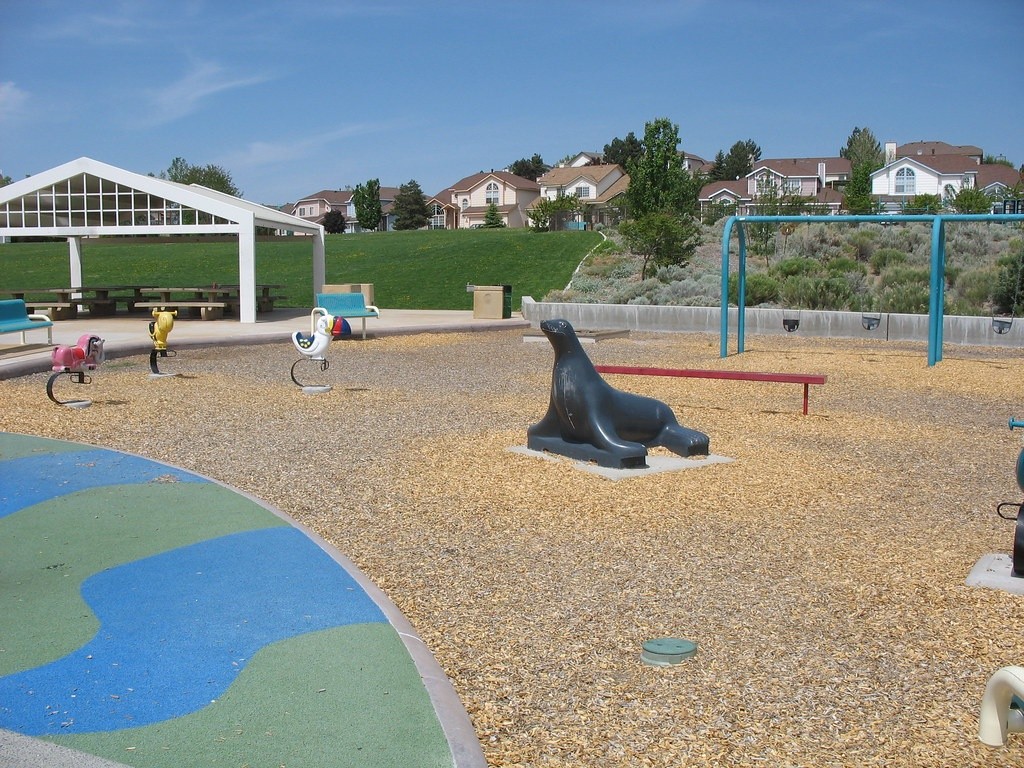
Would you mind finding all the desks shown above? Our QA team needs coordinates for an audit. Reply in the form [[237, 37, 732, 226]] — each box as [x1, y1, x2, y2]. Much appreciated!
[[0, 285, 280, 302]]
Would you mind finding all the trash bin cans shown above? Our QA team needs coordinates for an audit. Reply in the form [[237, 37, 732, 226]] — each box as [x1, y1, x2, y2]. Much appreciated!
[[473, 284, 512, 319]]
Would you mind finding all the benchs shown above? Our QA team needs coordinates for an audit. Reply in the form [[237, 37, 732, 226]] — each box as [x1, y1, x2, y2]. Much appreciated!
[[594, 365, 829, 415], [310, 293, 380, 340], [0, 295, 287, 347]]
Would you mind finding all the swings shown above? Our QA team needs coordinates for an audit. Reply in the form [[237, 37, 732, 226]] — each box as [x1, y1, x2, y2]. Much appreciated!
[[774, 221, 813, 332], [986, 221, 1024, 335], [855, 221, 893, 330]]
